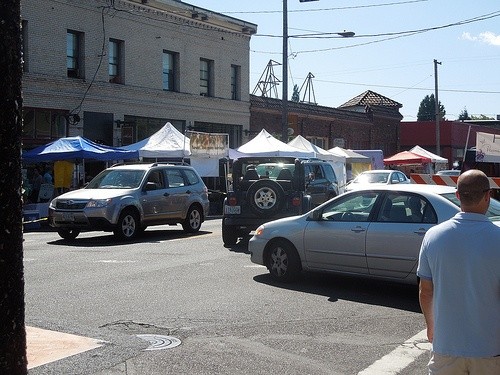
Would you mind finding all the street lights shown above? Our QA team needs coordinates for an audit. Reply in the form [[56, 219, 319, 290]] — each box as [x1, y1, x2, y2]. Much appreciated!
[[282, 0, 355, 144]]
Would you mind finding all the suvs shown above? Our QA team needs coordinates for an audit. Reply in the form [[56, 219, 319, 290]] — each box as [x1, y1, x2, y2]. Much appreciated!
[[222, 156, 340, 245], [47, 160, 209, 245]]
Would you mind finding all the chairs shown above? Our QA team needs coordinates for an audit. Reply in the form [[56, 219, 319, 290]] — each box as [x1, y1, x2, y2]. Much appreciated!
[[246, 169, 259, 180], [276, 168, 292, 180], [391, 206, 406, 222]]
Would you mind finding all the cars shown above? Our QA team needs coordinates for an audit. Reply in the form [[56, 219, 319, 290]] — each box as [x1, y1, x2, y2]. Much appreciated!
[[248, 183, 500, 294], [344, 170, 410, 195]]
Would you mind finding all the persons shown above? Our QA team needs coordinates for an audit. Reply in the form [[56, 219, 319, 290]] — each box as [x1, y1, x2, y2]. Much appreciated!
[[32, 171, 42, 201], [416, 170, 500, 375], [43, 169, 53, 184]]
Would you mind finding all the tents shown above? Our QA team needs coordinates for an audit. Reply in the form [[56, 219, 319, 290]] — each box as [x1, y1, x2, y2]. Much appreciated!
[[287, 135, 346, 195], [410, 144, 448, 173], [327, 146, 374, 185], [22, 135, 139, 189], [228, 128, 316, 160], [121, 121, 229, 192], [383, 151, 432, 174]]
